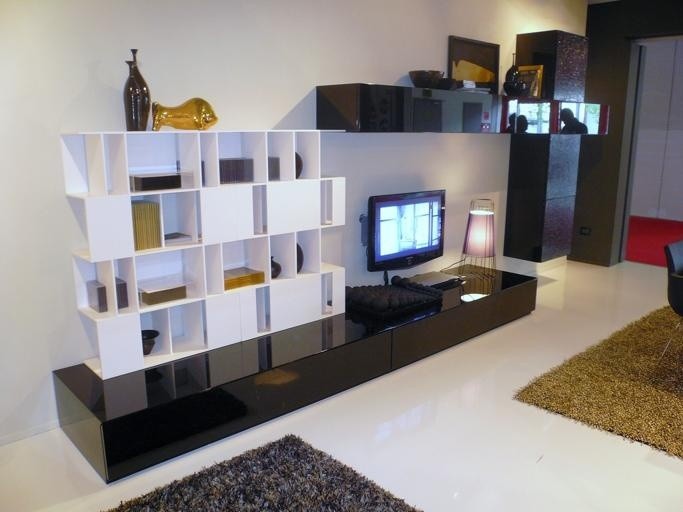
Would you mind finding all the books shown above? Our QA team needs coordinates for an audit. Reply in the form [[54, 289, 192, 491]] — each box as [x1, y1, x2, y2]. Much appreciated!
[[130, 173, 181, 192], [135, 203, 160, 251], [220, 158, 279, 184]]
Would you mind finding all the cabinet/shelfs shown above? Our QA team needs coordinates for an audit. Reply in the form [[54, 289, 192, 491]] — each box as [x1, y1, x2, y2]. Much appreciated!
[[318, 78, 518, 136], [54, 263, 537, 482], [65, 131, 347, 378]]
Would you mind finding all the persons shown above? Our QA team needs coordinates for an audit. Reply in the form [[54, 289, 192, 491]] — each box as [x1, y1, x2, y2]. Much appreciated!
[[560, 108, 588, 134], [506, 113, 530, 135]]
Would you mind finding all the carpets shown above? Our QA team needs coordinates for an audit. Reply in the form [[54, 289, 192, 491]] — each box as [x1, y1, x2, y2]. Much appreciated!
[[100, 433, 422, 512], [510, 304, 683, 461]]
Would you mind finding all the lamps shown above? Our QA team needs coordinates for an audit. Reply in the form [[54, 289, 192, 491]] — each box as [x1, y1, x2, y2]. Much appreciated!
[[461, 199, 497, 266]]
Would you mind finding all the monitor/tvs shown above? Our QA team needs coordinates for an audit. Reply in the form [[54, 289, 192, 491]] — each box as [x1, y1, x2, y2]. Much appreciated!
[[367, 189, 445, 272]]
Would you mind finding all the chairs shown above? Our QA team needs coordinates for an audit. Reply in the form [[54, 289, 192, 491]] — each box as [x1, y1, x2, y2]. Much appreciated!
[[658, 239, 683, 360]]
